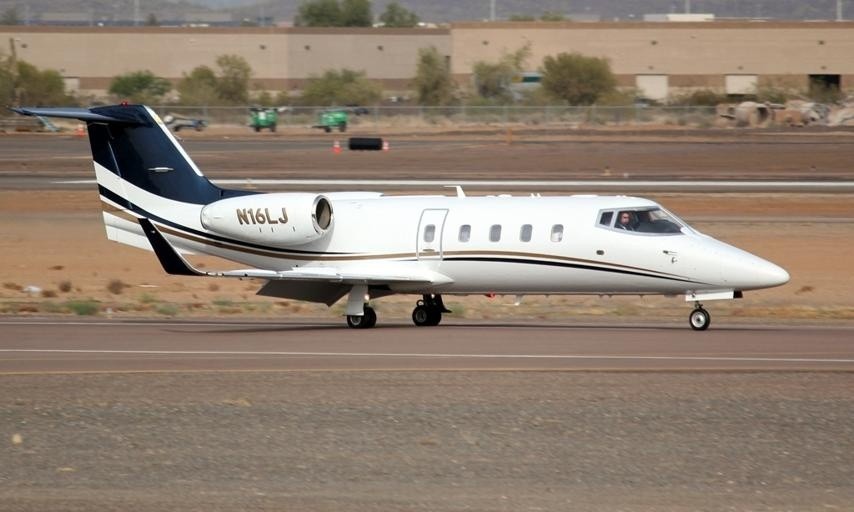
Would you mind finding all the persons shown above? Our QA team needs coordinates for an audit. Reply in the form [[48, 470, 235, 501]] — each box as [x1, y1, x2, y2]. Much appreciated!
[[615, 212, 635, 230]]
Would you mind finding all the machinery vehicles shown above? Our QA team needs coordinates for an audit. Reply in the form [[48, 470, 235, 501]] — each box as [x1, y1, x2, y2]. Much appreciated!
[[161, 107, 346, 134]]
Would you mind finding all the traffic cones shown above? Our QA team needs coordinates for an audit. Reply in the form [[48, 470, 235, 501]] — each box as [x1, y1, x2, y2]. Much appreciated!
[[334, 136, 343, 154], [381, 136, 390, 153]]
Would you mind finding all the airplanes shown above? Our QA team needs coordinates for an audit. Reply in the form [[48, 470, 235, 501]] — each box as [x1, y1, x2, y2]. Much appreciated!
[[5, 103, 790, 331]]
